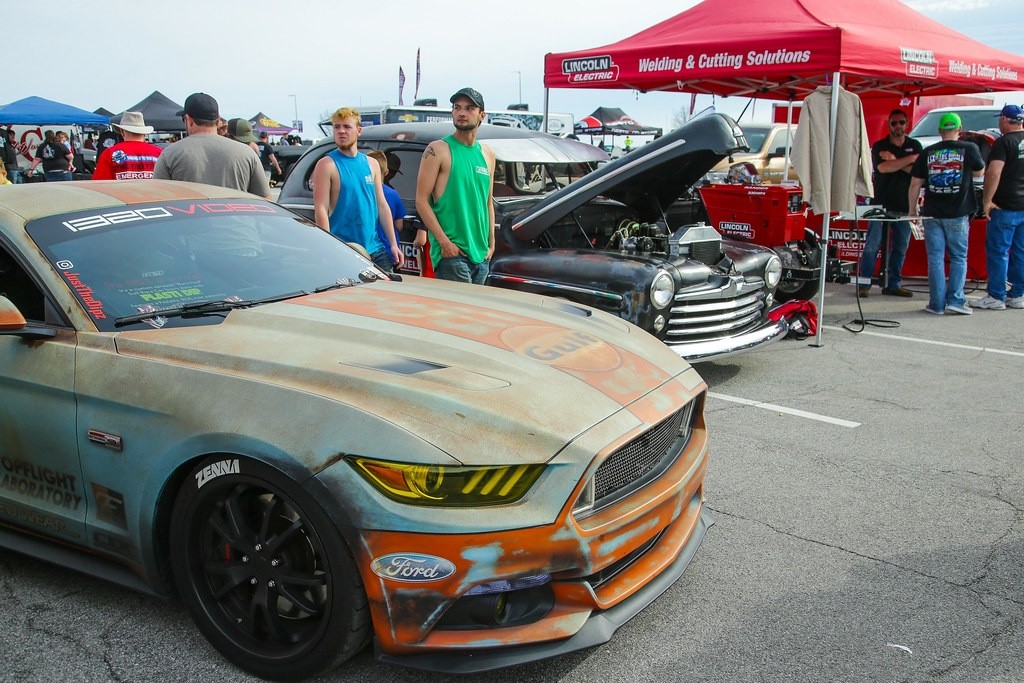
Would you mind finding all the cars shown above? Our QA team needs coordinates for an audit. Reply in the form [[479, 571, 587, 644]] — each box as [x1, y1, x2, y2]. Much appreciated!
[[301, 139, 320, 144], [711, 123, 799, 184], [908, 106, 1005, 149], [0, 179, 717, 683], [603, 145, 615, 154], [274, 112, 789, 363]]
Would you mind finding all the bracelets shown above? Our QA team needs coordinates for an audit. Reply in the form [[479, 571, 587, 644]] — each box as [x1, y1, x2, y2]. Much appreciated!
[[30, 168, 34, 173]]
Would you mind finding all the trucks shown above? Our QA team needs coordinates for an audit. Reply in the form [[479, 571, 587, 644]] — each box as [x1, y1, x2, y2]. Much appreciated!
[[318, 106, 574, 176], [2, 125, 97, 174]]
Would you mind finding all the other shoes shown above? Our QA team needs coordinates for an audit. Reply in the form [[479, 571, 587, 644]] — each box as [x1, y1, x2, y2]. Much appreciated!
[[859, 288, 869, 297], [882, 287, 913, 297]]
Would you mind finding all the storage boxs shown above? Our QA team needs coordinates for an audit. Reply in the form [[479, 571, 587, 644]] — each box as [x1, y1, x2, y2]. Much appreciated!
[[702, 180, 806, 244]]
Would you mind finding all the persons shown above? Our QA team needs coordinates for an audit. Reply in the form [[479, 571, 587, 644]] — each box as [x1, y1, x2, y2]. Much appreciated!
[[92, 112, 163, 181], [312, 107, 407, 274], [144, 135, 182, 144], [153, 92, 273, 285], [0, 130, 76, 184], [217, 118, 303, 187], [969, 105, 1024, 310], [85, 134, 98, 152], [383, 153, 403, 190], [598, 140, 605, 150], [908, 112, 985, 314], [416, 88, 495, 285], [857, 110, 923, 297], [625, 136, 637, 153]]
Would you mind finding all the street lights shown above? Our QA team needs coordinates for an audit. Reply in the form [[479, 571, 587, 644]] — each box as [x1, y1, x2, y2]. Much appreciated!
[[290, 95, 298, 129]]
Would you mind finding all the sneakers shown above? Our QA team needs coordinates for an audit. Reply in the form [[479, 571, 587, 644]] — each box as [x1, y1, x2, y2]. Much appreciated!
[[1005, 295, 1024, 308], [926, 304, 944, 314], [968, 295, 1006, 309], [945, 303, 972, 314]]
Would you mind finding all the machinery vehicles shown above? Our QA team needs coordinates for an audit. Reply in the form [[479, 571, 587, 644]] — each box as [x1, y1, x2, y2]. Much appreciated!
[[659, 162, 858, 304]]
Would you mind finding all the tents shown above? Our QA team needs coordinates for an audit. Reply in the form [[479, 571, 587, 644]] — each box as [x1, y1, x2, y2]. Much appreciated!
[[574, 107, 663, 151], [0, 91, 187, 143], [543, 0, 1024, 347], [248, 112, 299, 135]]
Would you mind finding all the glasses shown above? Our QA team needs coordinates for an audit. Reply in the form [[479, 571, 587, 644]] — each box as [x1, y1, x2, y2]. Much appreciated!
[[889, 119, 908, 126], [182, 115, 186, 122]]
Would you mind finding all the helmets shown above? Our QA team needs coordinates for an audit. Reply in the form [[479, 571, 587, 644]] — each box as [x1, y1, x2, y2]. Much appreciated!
[[727, 161, 762, 183]]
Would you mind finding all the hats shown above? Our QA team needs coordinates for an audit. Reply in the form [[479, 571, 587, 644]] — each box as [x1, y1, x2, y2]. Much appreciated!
[[220, 118, 228, 127], [228, 118, 260, 141], [112, 111, 153, 133], [993, 105, 1024, 120], [386, 153, 404, 174], [450, 87, 484, 110], [939, 113, 961, 129], [175, 93, 219, 120]]
